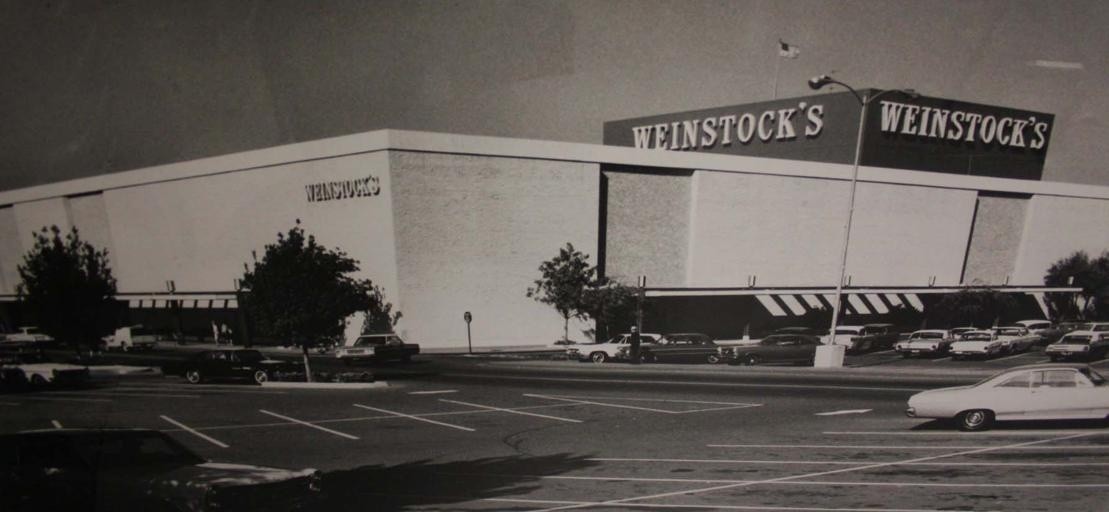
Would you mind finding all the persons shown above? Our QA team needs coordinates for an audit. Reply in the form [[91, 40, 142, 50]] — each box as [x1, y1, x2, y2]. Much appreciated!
[[629, 325, 640, 365], [210, 319, 234, 347]]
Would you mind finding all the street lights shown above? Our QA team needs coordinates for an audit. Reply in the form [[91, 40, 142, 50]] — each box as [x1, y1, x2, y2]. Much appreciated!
[[807, 75, 916, 341]]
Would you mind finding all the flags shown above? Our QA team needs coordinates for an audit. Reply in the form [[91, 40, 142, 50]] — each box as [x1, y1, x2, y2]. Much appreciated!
[[777, 40, 801, 60]]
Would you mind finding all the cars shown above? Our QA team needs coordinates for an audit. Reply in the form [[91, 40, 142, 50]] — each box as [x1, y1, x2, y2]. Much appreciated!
[[577, 326, 822, 367], [3, 326, 54, 342], [338, 334, 419, 362], [895, 319, 1109, 361], [906, 361, 1109, 430], [0, 362, 89, 388], [820, 321, 893, 356], [180, 349, 287, 385]]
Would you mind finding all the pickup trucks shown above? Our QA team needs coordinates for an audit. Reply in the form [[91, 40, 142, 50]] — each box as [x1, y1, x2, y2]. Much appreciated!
[[104, 325, 159, 353]]
